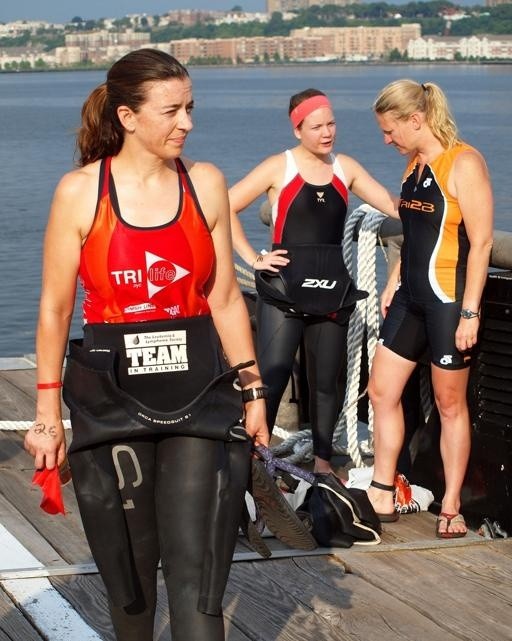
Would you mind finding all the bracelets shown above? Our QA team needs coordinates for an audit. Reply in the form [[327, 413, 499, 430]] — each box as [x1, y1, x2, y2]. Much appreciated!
[[38, 381, 64, 391], [459, 309, 480, 320], [252, 255, 263, 271], [242, 384, 273, 403]]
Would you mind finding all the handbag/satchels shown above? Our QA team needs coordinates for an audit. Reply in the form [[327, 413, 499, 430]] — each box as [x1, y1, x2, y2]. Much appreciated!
[[299, 471, 381, 548]]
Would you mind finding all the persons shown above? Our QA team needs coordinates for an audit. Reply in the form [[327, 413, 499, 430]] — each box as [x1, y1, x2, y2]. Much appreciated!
[[363, 78, 496, 541], [22, 47, 274, 641], [228, 88, 401, 483]]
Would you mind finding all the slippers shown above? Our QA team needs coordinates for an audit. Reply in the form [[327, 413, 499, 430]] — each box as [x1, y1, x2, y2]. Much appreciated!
[[238, 451, 317, 560], [377, 511, 399, 523], [437, 504, 467, 538]]
